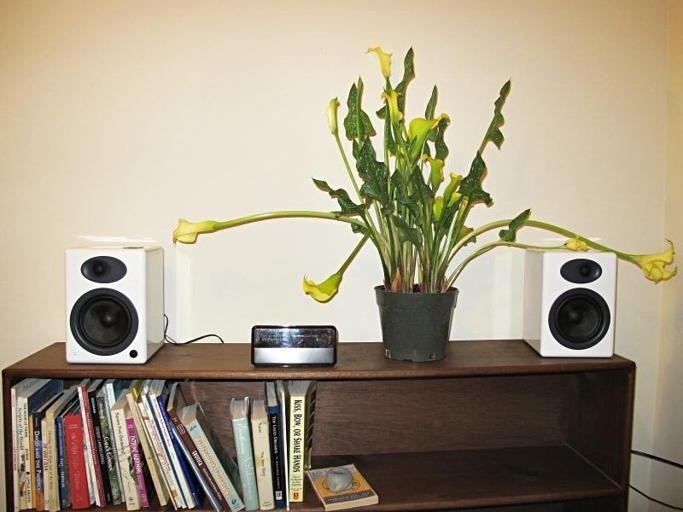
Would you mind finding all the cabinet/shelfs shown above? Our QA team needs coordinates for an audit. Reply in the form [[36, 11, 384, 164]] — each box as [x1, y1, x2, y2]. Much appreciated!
[[5, 338, 636, 510]]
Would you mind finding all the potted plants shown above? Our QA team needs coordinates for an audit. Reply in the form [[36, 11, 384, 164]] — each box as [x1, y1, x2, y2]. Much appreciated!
[[166, 47, 678, 362]]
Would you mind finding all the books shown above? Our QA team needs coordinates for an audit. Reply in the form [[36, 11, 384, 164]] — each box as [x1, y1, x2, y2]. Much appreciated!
[[303, 463, 379, 510], [5, 375, 319, 512]]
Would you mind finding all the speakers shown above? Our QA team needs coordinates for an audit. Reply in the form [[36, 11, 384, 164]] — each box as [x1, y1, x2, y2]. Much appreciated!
[[64, 243, 164, 365], [523, 248, 618, 358]]
